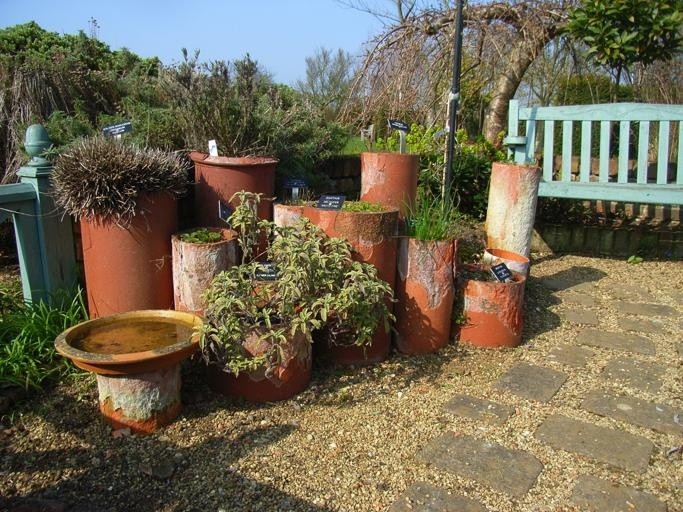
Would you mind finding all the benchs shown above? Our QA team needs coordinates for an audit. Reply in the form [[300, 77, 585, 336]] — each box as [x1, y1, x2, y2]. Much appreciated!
[[503, 98, 683, 204]]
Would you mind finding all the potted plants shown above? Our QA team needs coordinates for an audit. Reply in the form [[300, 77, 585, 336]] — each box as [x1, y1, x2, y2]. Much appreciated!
[[482, 155, 541, 279], [451, 263, 524, 346], [50, 133, 187, 316], [171, 225, 237, 322], [389, 187, 461, 357], [303, 198, 398, 365], [196, 188, 394, 400], [164, 45, 295, 270], [272, 189, 308, 247]]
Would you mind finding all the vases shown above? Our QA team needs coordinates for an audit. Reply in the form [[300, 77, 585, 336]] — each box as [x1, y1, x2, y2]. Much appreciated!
[[483, 248, 530, 281], [360, 152, 416, 233]]
